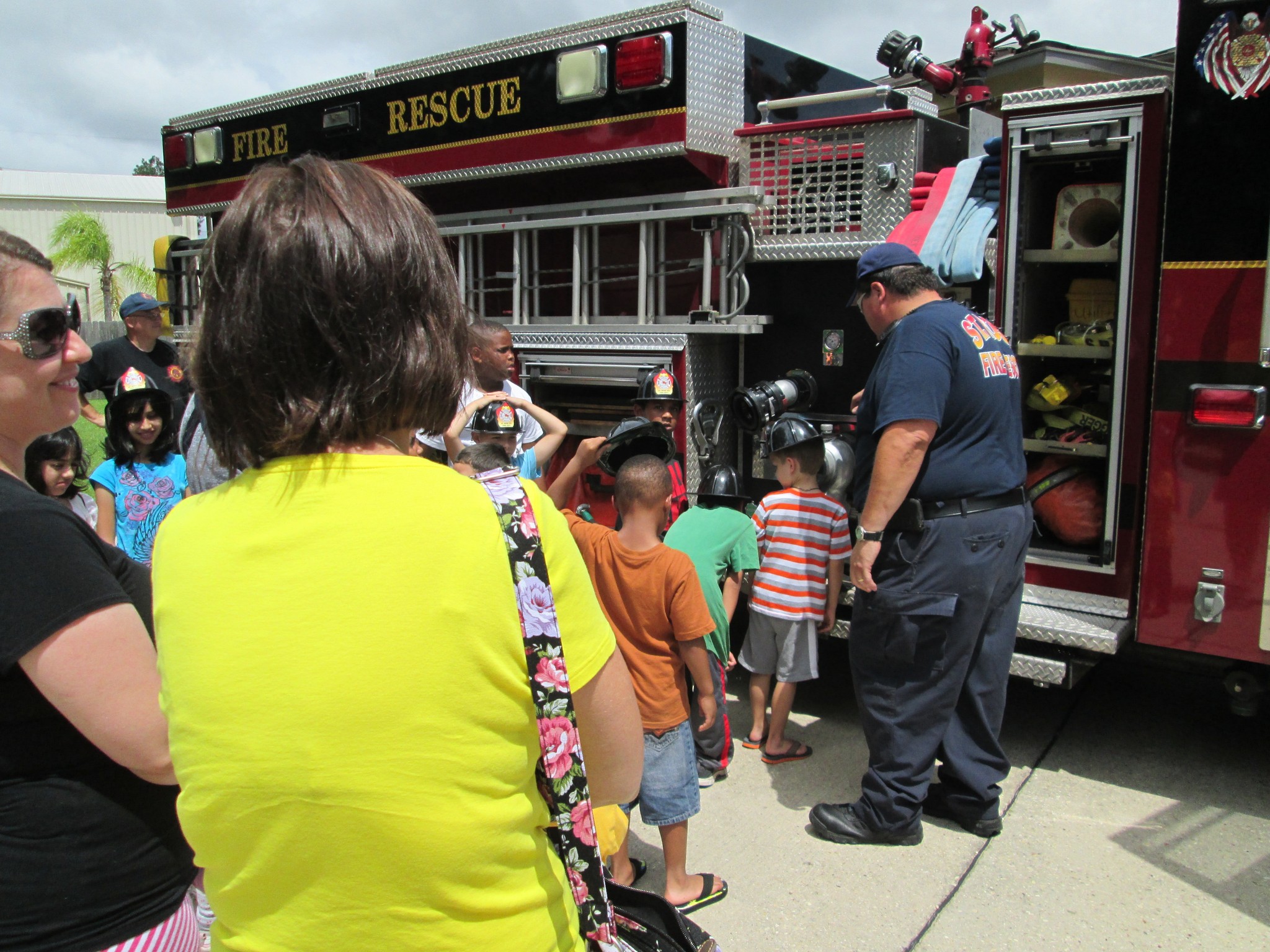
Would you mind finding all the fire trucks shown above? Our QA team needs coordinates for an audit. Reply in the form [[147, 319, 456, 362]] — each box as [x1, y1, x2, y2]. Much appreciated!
[[138, 0, 1269, 693]]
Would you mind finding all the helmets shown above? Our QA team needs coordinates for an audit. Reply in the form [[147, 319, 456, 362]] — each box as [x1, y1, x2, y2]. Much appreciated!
[[631, 368, 689, 403], [761, 416, 824, 459], [464, 400, 526, 433], [594, 416, 678, 476], [104, 366, 176, 411], [686, 463, 752, 499]]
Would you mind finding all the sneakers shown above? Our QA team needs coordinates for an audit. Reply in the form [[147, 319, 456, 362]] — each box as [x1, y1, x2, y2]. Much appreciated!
[[808, 803, 924, 847], [922, 781, 1003, 836]]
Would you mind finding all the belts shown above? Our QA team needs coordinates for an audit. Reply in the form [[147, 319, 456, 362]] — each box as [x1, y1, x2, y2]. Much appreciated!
[[924, 484, 1030, 520]]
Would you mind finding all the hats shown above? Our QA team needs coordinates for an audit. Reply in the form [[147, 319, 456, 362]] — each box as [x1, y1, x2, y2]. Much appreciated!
[[846, 242, 924, 307], [120, 292, 171, 319]]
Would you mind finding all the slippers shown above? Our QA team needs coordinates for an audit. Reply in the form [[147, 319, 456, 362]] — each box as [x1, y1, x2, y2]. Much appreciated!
[[761, 739, 813, 764], [674, 872, 729, 915], [743, 731, 769, 749], [609, 856, 646, 886]]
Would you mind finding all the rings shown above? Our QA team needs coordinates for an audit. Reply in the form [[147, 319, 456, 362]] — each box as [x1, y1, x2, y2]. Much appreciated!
[[857, 579, 864, 582]]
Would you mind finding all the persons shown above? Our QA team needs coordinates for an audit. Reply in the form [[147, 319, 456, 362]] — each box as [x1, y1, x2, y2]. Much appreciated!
[[442, 391, 568, 481], [615, 368, 689, 544], [662, 463, 760, 786], [410, 434, 424, 456], [88, 367, 195, 569], [807, 242, 1043, 846], [0, 231, 217, 952], [177, 392, 244, 495], [545, 416, 728, 916], [151, 153, 645, 952], [75, 292, 196, 456], [738, 417, 852, 764], [25, 425, 98, 534], [415, 319, 544, 473]]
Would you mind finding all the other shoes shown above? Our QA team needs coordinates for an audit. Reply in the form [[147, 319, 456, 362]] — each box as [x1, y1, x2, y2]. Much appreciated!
[[693, 762, 730, 785]]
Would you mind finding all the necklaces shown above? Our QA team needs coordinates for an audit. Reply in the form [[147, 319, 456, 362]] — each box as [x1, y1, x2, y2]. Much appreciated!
[[792, 486, 821, 491], [376, 434, 402, 452]]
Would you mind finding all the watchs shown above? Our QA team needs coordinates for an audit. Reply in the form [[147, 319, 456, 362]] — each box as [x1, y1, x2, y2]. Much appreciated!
[[855, 525, 885, 541]]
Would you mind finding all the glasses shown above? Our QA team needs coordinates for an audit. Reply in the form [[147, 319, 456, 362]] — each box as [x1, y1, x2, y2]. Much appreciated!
[[0, 292, 82, 359], [856, 290, 867, 314], [129, 311, 165, 324]]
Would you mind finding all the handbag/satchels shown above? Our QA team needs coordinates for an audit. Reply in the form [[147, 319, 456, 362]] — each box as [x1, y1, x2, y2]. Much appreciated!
[[544, 824, 722, 952]]
